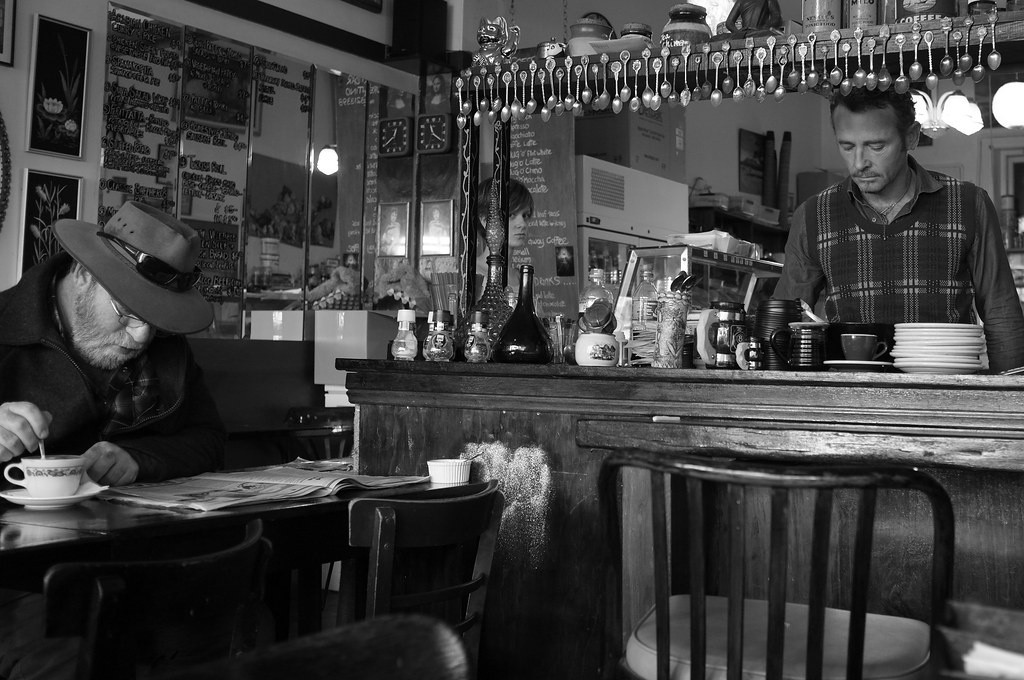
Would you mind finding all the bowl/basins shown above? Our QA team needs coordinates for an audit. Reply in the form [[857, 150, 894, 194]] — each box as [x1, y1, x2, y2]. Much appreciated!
[[825, 319, 890, 358], [659, 3, 712, 50], [568, 12, 617, 57], [428, 458, 473, 483]]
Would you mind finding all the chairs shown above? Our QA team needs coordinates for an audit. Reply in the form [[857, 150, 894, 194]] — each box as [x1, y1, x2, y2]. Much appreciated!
[[595, 450, 955, 680], [9, 428, 504, 680]]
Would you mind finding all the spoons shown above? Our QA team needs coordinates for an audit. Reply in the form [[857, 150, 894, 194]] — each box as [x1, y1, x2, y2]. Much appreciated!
[[455, 56, 592, 130], [594, 36, 778, 114], [670, 270, 696, 292], [774, 13, 1000, 104]]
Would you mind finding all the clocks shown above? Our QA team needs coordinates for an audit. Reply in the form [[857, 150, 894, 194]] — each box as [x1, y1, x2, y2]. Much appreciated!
[[379, 112, 452, 158]]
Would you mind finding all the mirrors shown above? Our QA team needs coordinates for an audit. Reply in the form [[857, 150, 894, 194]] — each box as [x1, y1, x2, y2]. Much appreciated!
[[240, 46, 369, 340]]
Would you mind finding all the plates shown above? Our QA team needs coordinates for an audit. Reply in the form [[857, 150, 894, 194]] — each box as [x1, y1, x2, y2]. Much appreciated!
[[589, 38, 648, 55], [0, 489, 93, 509], [889, 322, 986, 375], [823, 360, 893, 372]]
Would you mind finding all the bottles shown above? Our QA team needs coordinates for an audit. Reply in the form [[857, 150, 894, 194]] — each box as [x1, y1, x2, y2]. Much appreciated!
[[630, 271, 658, 368], [577, 269, 612, 337], [464, 311, 490, 364], [544, 317, 576, 364], [489, 265, 552, 365], [422, 309, 455, 362], [391, 309, 418, 361]]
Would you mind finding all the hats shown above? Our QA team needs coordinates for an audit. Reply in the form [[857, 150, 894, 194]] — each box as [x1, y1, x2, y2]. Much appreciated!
[[52, 201, 214, 335]]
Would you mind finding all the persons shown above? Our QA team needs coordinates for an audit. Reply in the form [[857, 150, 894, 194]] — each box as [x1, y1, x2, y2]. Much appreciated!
[[768, 67, 1024, 375], [0, 201, 276, 680], [476, 176, 542, 313]]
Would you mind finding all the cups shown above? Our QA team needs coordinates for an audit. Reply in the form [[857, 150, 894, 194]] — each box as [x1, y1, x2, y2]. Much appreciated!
[[652, 292, 693, 369], [771, 320, 830, 370], [4, 455, 85, 497], [574, 333, 619, 367], [841, 334, 887, 360]]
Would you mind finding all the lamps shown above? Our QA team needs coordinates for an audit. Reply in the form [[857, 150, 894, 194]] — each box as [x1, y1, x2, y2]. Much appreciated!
[[992, 81, 1024, 132], [317, 144, 339, 176], [908, 87, 982, 137]]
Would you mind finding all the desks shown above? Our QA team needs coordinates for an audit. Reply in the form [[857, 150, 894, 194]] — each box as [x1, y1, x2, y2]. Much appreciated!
[[0, 479, 488, 647], [247, 291, 302, 311], [189, 336, 314, 469]]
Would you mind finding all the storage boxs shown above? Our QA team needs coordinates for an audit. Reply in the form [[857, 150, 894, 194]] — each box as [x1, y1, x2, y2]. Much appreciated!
[[577, 97, 685, 183], [250, 309, 315, 340], [689, 193, 783, 226], [314, 307, 397, 408], [796, 171, 845, 202]]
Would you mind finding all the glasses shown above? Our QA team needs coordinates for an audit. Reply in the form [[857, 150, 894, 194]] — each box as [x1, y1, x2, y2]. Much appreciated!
[[110, 299, 149, 328], [95, 231, 204, 294]]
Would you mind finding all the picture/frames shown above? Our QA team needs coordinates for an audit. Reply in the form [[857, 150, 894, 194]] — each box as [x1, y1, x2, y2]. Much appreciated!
[[0, 0, 18, 68], [17, 168, 84, 284], [739, 128, 764, 194], [25, 11, 93, 161], [377, 202, 410, 259], [419, 199, 454, 257]]
